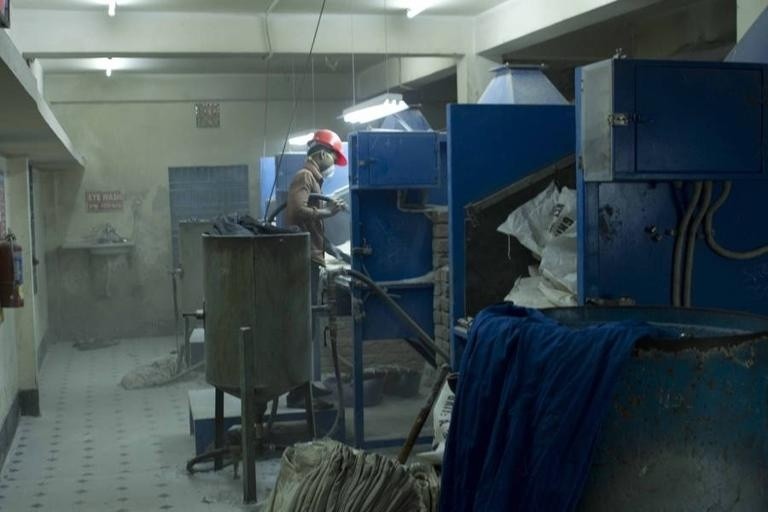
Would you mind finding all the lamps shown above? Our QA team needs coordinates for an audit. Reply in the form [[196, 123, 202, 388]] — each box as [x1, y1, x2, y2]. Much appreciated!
[[335, 0, 410, 125], [288, 54, 319, 147]]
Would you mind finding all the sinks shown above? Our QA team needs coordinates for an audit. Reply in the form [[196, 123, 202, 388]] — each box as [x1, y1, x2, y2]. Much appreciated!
[[93, 241, 135, 254]]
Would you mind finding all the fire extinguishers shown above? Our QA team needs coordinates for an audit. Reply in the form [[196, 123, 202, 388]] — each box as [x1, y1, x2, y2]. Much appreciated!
[[0, 228, 25, 308]]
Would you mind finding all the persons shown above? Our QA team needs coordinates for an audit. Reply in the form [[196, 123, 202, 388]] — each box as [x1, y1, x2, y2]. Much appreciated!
[[288, 128, 351, 413]]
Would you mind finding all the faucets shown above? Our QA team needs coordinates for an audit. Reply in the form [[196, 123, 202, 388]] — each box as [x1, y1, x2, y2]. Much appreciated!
[[103, 222, 114, 240]]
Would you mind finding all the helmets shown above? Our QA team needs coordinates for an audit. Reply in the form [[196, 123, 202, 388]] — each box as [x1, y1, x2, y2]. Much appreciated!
[[308, 129, 347, 166]]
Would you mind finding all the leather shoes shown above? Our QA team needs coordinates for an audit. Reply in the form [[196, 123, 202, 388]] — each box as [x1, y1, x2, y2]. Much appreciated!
[[287, 384, 334, 409]]
